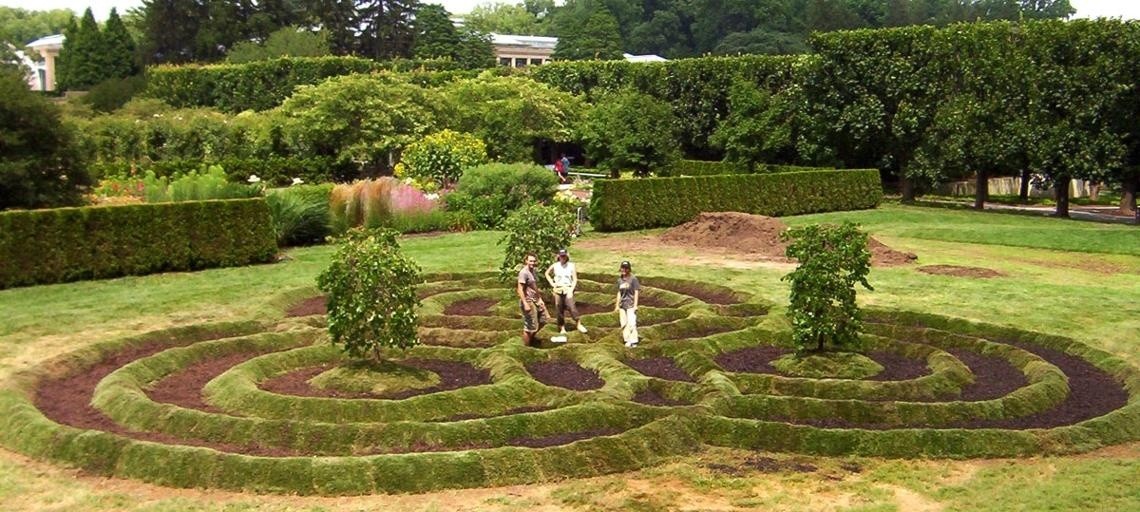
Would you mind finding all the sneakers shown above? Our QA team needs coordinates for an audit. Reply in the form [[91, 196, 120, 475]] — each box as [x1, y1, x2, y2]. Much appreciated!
[[558, 324, 588, 336], [624, 341, 638, 348]]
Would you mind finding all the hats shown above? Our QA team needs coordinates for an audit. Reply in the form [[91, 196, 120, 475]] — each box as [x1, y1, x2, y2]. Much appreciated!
[[558, 249, 569, 259], [620, 261, 633, 271]]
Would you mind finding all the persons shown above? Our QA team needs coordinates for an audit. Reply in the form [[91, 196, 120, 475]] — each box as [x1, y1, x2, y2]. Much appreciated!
[[560, 151, 570, 178], [553, 158, 564, 184], [421, 178, 456, 201], [612, 260, 641, 348], [544, 248, 589, 333], [517, 253, 550, 347]]
